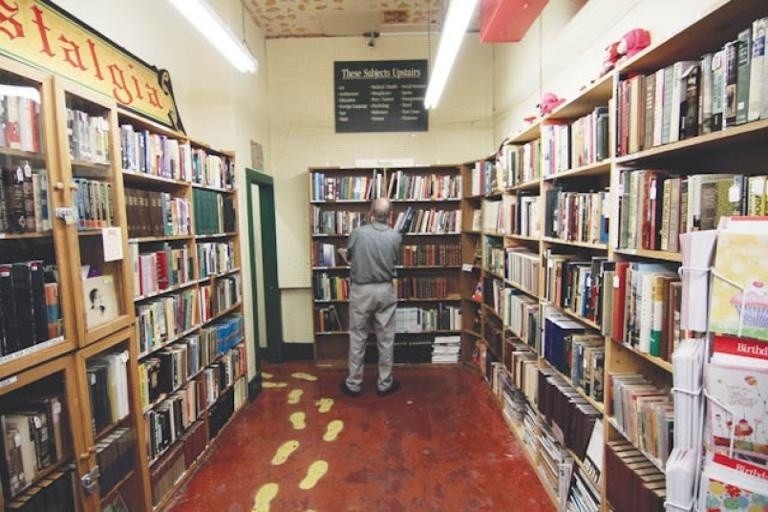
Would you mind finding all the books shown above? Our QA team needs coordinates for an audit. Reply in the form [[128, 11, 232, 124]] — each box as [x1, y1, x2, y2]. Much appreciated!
[[1, 83, 251, 512], [469, 16, 767, 512], [310, 167, 462, 363]]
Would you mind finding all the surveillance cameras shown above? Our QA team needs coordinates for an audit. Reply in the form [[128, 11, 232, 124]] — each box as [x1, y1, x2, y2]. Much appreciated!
[[367, 40, 375, 48]]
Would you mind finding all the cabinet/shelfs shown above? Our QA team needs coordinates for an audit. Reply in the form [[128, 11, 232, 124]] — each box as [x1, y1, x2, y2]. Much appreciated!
[[0, 57, 250, 511], [307, 166, 464, 369], [462, 0, 768, 512]]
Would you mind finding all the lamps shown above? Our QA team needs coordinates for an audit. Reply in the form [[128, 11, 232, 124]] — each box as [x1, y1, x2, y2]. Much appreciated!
[[422, 0, 478, 109], [363, 32, 380, 49], [173, 0, 258, 74]]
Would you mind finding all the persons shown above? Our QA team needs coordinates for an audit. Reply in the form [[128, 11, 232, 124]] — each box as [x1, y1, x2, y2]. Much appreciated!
[[341, 197, 406, 396]]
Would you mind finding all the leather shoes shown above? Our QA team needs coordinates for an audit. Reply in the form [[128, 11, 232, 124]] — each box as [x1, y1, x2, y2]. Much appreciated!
[[379, 380, 399, 396], [342, 379, 361, 397]]
